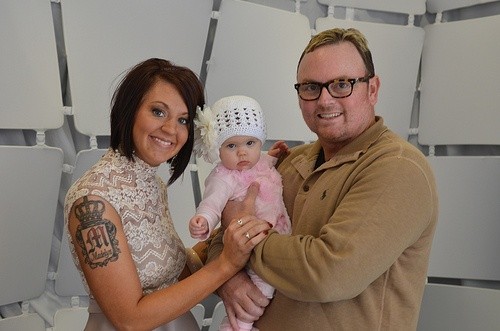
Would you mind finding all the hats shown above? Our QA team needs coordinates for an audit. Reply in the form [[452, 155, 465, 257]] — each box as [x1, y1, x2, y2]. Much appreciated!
[[193, 95, 266, 162]]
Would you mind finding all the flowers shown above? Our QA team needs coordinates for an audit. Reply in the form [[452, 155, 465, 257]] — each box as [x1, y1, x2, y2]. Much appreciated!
[[193, 104, 220, 164]]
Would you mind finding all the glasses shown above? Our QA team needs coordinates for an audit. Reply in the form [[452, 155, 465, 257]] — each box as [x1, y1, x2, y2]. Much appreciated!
[[293, 76, 367, 101]]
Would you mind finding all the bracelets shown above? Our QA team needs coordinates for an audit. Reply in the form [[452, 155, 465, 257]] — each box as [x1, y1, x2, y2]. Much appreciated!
[[183, 248, 204, 274]]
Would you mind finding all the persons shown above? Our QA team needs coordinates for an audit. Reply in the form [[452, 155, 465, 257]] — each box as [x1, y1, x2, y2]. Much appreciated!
[[63, 56, 273, 331], [188, 94, 293, 331], [206, 28, 438, 331]]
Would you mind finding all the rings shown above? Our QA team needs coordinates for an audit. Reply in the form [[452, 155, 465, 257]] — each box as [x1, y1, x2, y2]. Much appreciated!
[[245, 231, 249, 239], [237, 218, 243, 226]]
